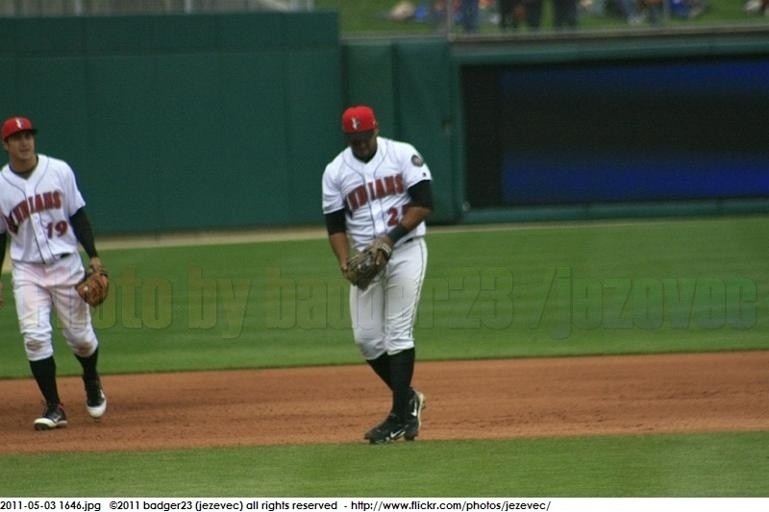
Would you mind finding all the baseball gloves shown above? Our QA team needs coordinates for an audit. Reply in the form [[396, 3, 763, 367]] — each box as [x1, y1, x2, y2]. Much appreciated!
[[74, 264, 109, 305], [343, 237, 393, 289]]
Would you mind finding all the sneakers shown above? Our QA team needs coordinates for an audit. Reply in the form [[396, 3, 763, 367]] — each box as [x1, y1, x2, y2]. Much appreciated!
[[34, 402, 68, 430], [82, 373, 107, 417], [363, 387, 426, 444]]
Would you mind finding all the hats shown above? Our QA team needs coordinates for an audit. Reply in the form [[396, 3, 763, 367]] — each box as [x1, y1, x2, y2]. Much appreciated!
[[2, 116, 38, 141], [342, 105, 376, 143]]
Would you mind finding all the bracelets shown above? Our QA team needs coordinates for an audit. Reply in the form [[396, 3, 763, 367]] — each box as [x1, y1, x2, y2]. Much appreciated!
[[387, 223, 407, 243]]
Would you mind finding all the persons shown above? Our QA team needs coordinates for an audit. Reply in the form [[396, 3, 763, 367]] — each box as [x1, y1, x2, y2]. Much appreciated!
[[320, 105, 434, 442], [0, 116, 108, 430]]
